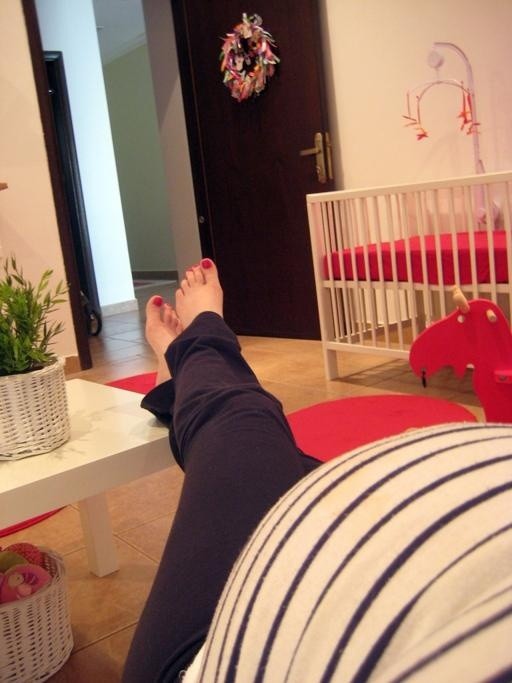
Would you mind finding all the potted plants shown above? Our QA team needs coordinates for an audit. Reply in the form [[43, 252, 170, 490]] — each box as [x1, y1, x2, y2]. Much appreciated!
[[0, 253, 73, 461]]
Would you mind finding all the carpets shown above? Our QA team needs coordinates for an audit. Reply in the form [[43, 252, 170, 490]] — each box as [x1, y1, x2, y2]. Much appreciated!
[[0, 378, 183, 578], [1, 370, 159, 537], [287, 393, 478, 464]]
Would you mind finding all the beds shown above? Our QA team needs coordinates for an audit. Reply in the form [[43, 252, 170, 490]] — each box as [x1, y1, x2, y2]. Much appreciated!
[[304, 170, 511, 388]]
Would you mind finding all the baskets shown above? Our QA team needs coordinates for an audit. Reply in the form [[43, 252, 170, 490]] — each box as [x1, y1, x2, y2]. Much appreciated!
[[0, 354, 72, 462], [0, 544, 74, 682]]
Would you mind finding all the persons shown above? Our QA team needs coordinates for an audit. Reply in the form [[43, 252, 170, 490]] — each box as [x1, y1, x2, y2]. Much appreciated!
[[118, 252, 510, 682]]
